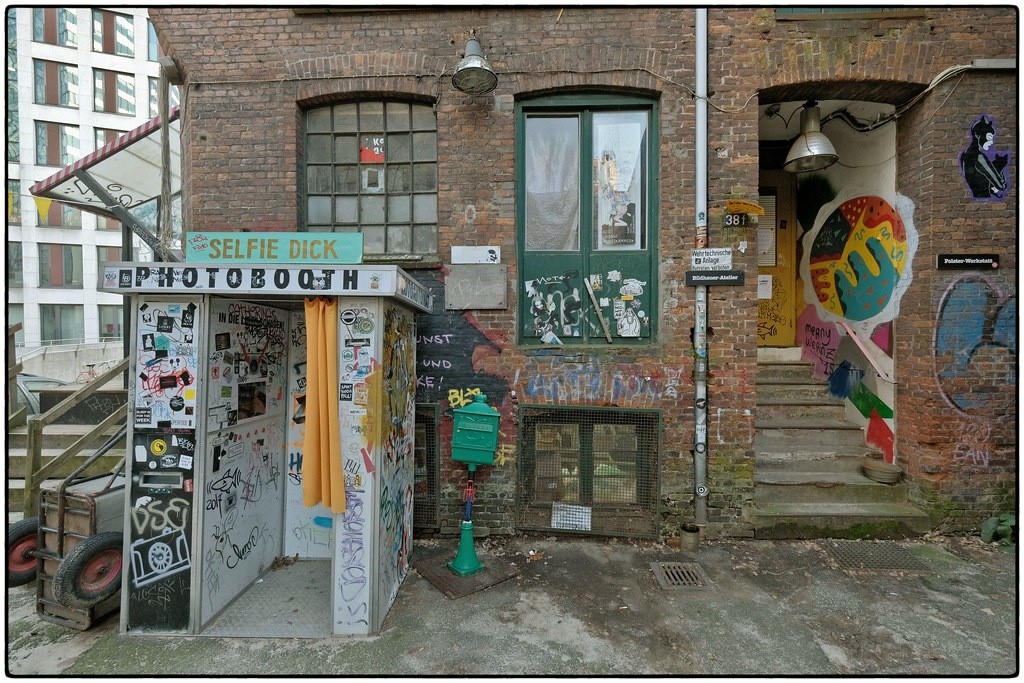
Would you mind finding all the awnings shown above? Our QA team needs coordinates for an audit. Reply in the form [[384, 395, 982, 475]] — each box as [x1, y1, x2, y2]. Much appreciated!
[[30, 103, 183, 261]]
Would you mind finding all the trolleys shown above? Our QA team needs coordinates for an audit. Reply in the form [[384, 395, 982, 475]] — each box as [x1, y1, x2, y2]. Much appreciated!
[[6, 423, 126, 632]]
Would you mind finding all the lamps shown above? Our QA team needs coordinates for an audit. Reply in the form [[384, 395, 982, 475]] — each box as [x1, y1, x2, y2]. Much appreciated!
[[783, 100, 839, 174], [452, 30, 499, 95]]
